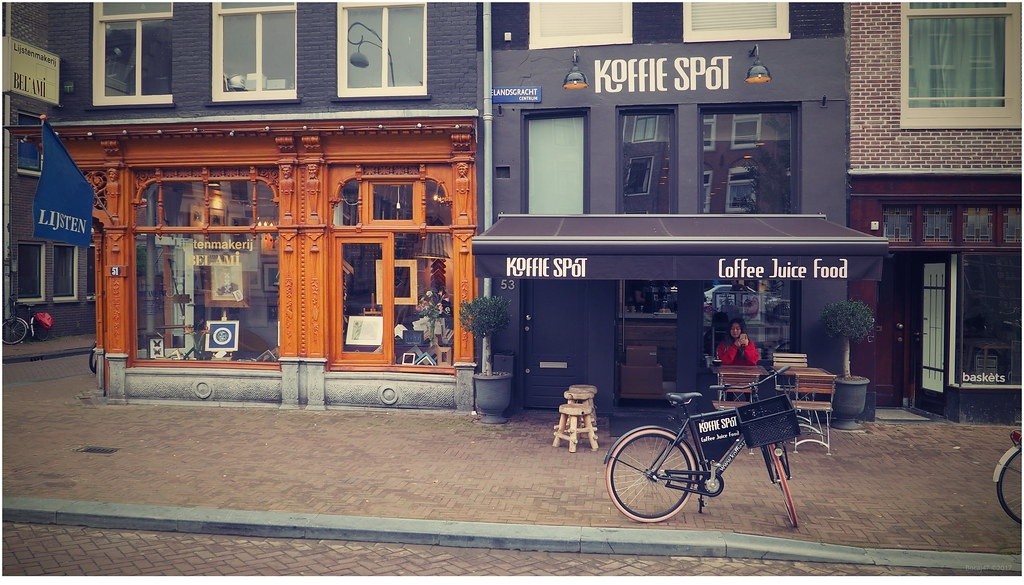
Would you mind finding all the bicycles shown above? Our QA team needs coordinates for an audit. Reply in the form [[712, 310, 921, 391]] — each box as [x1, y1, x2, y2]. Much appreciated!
[[2, 294, 51, 345], [88, 338, 98, 375], [601, 365, 803, 529], [993, 420, 1021, 525]]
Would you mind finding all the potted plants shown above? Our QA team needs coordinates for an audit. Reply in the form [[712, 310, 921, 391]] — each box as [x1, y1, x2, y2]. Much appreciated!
[[821, 298, 878, 430], [460, 294, 513, 424]]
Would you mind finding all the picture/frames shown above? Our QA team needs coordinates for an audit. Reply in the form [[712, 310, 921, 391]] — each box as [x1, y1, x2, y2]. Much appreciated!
[[204, 253, 249, 307], [206, 320, 239, 352], [146, 337, 165, 360]]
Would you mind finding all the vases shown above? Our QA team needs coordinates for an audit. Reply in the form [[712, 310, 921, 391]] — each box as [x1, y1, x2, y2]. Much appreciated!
[[193, 335, 205, 360], [430, 320, 436, 346]]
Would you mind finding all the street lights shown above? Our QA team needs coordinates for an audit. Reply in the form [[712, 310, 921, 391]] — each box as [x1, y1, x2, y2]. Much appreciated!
[[347, 21, 396, 87]]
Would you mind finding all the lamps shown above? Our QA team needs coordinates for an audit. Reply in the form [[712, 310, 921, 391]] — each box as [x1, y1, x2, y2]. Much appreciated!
[[563, 49, 588, 89], [396, 187, 401, 209], [348, 21, 396, 88], [743, 44, 771, 82]]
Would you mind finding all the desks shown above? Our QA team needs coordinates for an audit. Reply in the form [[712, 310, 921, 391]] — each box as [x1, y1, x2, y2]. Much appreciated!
[[958, 338, 1011, 382], [712, 365, 833, 430]]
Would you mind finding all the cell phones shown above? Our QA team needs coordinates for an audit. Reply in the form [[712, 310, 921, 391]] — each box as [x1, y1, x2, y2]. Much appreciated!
[[740, 334, 746, 339]]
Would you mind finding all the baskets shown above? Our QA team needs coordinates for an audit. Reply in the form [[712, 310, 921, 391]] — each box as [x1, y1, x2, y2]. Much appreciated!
[[736, 394, 802, 448]]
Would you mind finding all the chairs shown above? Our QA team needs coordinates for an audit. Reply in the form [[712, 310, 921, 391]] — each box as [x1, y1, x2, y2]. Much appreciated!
[[712, 352, 838, 454]]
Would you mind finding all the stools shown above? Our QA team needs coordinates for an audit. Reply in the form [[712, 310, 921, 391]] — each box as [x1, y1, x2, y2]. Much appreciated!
[[552, 384, 598, 451]]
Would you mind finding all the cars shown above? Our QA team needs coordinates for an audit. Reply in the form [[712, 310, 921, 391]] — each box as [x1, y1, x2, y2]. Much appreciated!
[[703, 283, 759, 306]]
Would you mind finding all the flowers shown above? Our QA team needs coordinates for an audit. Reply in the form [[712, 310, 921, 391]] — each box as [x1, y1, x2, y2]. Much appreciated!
[[415, 291, 452, 341], [185, 321, 210, 354]]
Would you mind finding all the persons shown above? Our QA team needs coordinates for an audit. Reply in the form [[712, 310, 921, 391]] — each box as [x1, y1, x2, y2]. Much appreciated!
[[717, 319, 761, 402], [395, 292, 453, 364]]
